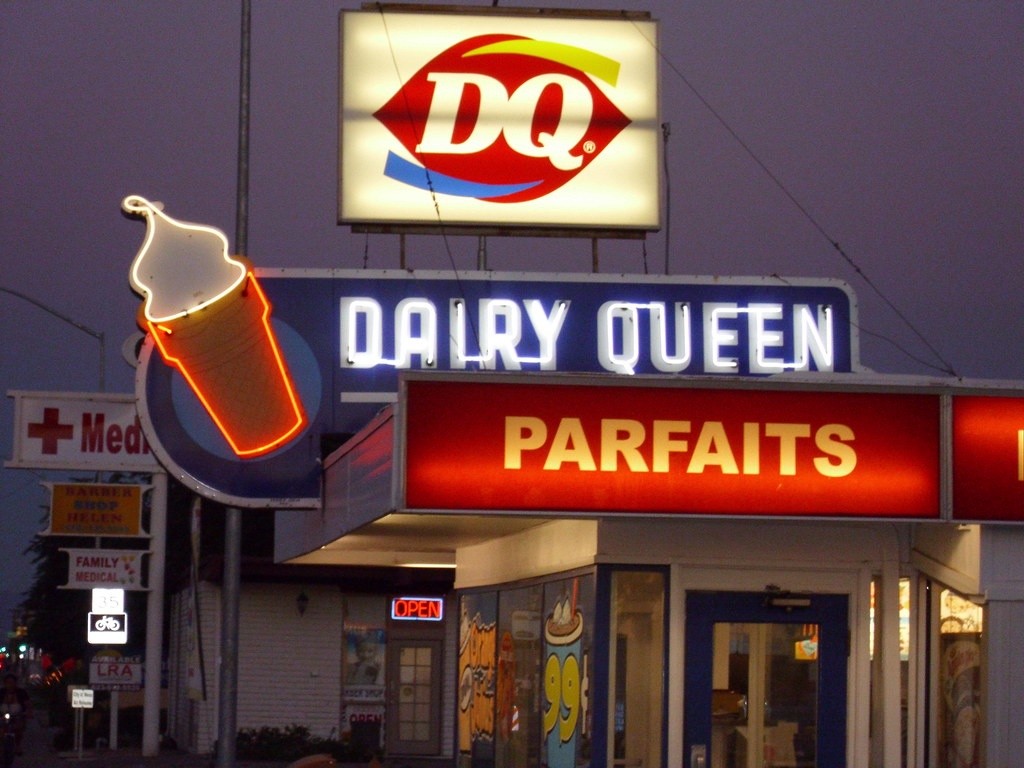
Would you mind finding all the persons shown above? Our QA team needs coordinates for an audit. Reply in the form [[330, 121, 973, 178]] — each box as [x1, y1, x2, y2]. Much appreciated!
[[0, 674, 33, 756], [346, 638, 379, 685]]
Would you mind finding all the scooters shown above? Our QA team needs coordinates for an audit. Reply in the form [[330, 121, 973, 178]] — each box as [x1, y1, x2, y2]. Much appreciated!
[[0, 710, 29, 768]]
